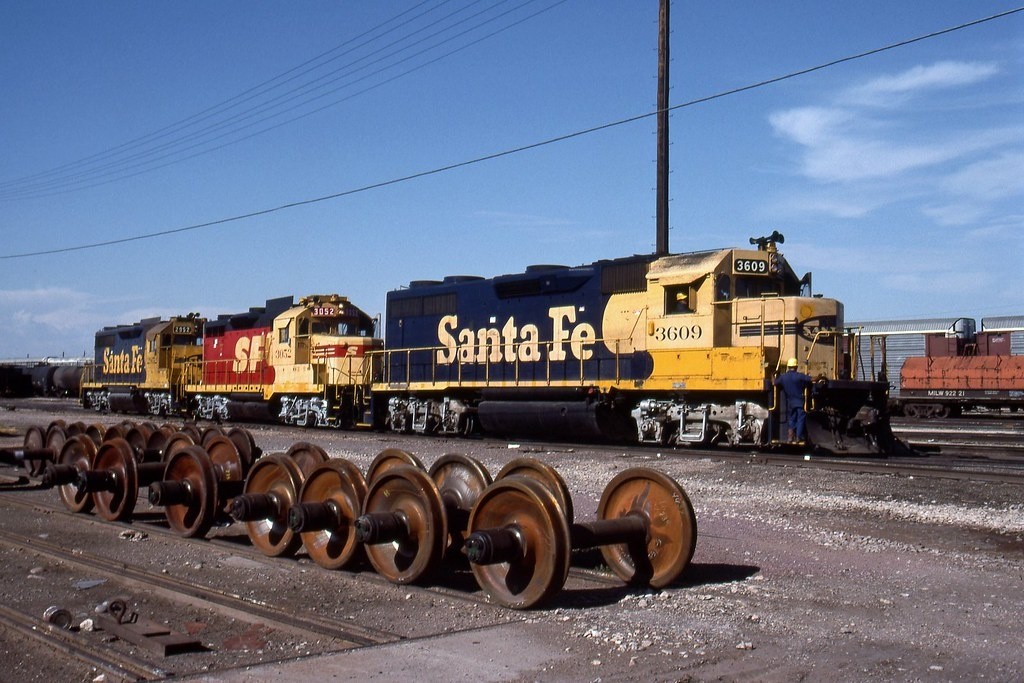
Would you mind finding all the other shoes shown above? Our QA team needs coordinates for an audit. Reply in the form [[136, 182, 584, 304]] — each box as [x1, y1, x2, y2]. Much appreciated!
[[787, 437, 793, 442], [796, 436, 803, 443]]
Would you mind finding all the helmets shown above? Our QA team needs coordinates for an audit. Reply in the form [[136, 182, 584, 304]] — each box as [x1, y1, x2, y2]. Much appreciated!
[[787, 358, 798, 367]]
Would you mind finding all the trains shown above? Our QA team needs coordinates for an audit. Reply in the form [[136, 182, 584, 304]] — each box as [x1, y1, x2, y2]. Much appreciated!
[[79, 230, 917, 457]]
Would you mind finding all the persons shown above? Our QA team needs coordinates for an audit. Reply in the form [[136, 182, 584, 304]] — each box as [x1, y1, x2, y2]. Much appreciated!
[[772, 358, 816, 443]]
